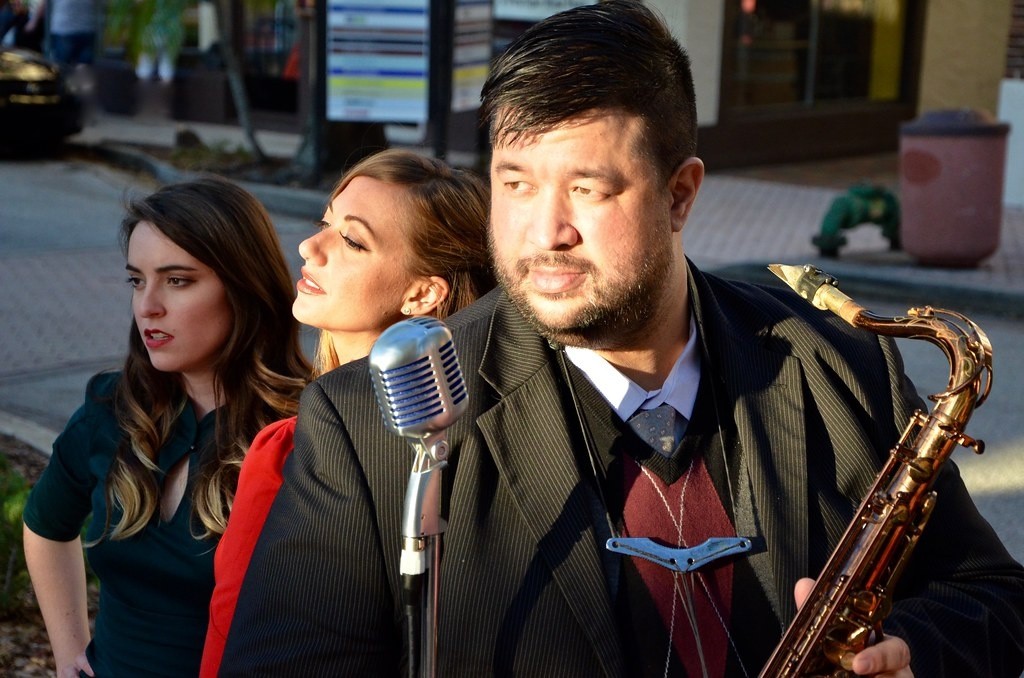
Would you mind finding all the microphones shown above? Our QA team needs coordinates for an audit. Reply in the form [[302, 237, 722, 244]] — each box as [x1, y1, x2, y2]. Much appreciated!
[[369, 316, 469, 602]]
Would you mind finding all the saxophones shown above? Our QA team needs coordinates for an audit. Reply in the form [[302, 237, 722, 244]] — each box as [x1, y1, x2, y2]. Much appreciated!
[[756, 255, 997, 678]]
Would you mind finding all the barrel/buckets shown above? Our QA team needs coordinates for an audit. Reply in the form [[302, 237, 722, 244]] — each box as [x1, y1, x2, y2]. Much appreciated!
[[902, 124, 1009, 263]]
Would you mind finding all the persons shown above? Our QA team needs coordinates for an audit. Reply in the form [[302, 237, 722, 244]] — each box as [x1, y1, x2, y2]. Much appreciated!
[[213, 0, 1024, 678], [198, 144, 500, 678], [19, 176, 314, 678]]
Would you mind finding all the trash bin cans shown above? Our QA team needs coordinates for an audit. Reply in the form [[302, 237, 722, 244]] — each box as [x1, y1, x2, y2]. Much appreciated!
[[898, 108, 1011, 269]]
